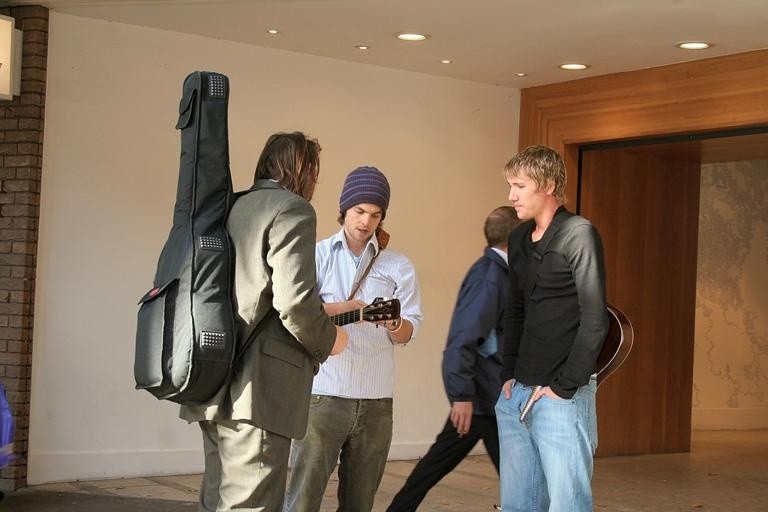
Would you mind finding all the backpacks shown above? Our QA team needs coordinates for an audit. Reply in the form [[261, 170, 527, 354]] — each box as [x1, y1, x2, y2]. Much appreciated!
[[134, 71, 285, 405]]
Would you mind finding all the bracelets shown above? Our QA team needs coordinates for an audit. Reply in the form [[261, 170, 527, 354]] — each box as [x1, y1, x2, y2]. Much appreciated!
[[390, 317, 403, 333]]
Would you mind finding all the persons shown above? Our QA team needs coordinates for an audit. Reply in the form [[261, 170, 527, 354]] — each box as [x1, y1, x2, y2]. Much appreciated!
[[177, 129, 349, 512], [283, 165, 423, 511], [384, 206, 531, 511], [494, 142, 610, 512]]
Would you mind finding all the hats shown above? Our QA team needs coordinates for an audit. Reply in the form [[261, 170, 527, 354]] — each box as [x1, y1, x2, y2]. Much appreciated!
[[339, 166, 390, 214]]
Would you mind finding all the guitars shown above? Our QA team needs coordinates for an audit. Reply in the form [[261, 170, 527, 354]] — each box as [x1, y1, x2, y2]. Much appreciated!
[[325, 299, 400, 329]]
